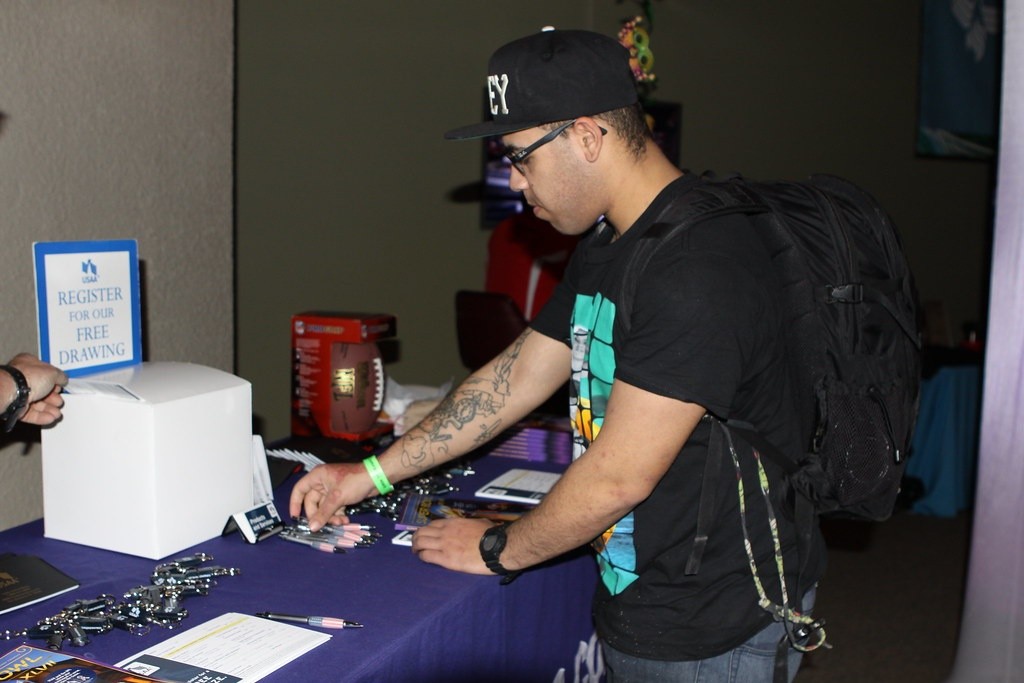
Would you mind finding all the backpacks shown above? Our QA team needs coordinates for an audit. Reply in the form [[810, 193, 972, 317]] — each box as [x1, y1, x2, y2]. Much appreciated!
[[619, 166, 934, 529]]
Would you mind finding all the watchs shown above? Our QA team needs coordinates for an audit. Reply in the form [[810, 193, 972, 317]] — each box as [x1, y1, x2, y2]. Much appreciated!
[[479, 522, 541, 585], [0, 364, 32, 435]]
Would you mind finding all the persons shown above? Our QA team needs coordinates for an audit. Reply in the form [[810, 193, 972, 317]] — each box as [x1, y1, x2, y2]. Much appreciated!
[[0, 353, 69, 436], [289, 27, 827, 683]]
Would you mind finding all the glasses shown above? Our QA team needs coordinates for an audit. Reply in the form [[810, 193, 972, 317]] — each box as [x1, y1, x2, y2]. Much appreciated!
[[502, 119, 608, 175]]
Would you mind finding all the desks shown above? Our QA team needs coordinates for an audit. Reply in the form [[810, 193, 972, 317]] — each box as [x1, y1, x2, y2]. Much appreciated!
[[0, 436, 608, 683]]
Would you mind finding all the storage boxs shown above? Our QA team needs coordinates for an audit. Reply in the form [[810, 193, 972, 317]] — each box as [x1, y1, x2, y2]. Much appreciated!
[[291, 310, 397, 464], [40, 361, 254, 563]]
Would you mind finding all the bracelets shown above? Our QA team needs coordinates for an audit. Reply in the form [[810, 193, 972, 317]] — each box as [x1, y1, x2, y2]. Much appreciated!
[[362, 455, 394, 496]]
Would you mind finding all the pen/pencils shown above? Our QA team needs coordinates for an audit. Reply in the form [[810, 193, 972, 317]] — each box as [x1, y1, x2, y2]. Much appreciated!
[[253, 611, 364, 631], [279, 517, 384, 555], [394, 522, 426, 531]]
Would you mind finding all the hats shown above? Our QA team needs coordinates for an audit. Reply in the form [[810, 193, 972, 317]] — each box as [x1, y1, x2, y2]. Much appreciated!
[[444, 24, 639, 141]]
